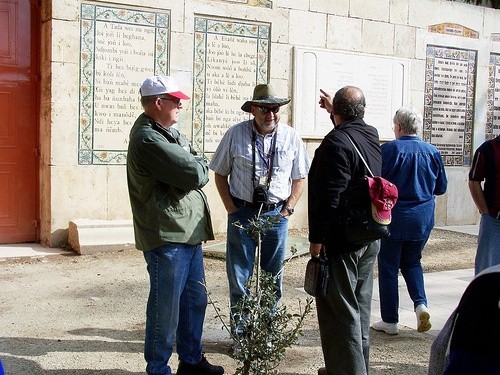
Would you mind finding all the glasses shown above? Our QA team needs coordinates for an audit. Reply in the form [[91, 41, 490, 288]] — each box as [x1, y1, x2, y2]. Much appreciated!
[[159, 98, 180, 104], [256, 106, 281, 114]]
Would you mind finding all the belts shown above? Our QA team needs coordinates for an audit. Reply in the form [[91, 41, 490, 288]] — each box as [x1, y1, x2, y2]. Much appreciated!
[[234, 197, 289, 211]]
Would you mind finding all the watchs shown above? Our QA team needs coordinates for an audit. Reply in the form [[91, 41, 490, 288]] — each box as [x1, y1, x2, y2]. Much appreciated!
[[285, 206, 295, 215]]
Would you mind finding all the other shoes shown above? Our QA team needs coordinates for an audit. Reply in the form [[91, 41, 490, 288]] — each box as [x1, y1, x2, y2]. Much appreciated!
[[372, 319, 400, 335], [177, 355, 224, 375], [416, 304, 432, 333]]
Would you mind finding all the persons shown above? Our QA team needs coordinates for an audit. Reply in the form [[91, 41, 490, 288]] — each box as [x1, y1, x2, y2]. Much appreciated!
[[209, 84, 310, 356], [307, 86, 385, 375], [469, 135, 500, 276], [371, 106, 448, 334], [126, 76, 224, 375]]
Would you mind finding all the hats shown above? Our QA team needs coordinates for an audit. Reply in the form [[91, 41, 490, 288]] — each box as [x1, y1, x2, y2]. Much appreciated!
[[140, 76, 192, 101], [241, 84, 291, 112]]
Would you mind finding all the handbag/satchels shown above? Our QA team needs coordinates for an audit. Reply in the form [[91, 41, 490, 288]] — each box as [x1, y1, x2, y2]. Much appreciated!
[[304, 259, 328, 296], [373, 175, 399, 209]]
